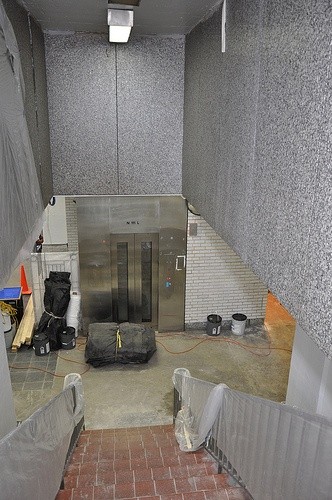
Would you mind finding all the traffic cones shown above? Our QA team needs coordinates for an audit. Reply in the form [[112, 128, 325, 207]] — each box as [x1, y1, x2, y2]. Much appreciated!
[[19, 264, 32, 295]]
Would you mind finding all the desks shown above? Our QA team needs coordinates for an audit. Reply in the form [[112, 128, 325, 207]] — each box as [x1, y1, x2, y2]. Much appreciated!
[[0, 286, 23, 308]]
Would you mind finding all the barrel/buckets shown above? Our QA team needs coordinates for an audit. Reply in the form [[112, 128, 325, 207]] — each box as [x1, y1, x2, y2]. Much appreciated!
[[4, 326, 15, 350], [33, 334, 50, 356], [231, 313, 248, 335], [11, 320, 17, 333], [207, 314, 222, 336], [59, 326, 76, 350]]
